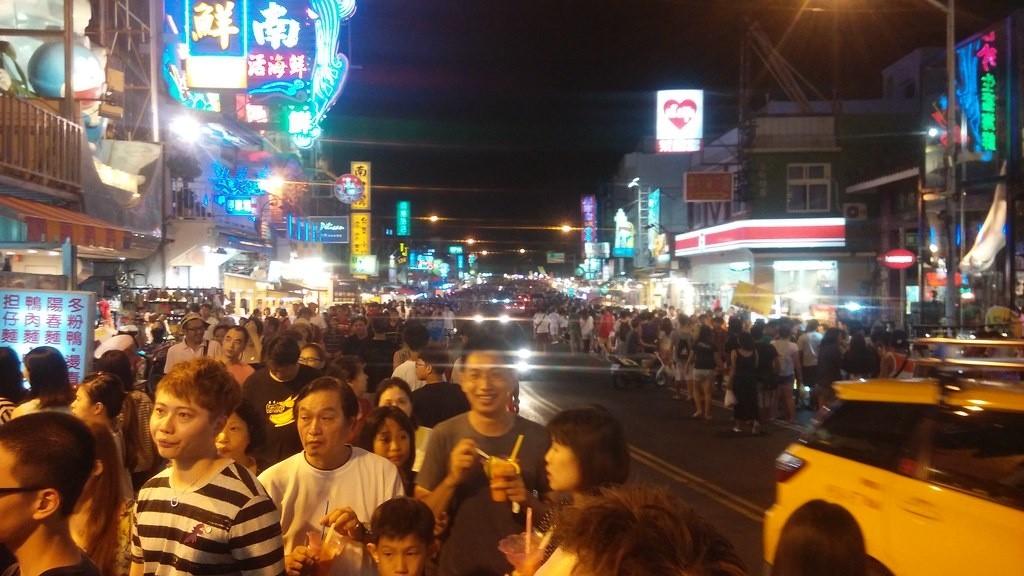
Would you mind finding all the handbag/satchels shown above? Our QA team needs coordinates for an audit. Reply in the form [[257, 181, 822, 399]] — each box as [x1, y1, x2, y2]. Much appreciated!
[[725, 390, 736, 407]]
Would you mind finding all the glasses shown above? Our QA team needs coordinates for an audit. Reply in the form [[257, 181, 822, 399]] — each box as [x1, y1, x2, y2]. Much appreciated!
[[416, 363, 426, 368], [188, 327, 204, 331], [298, 357, 320, 364]]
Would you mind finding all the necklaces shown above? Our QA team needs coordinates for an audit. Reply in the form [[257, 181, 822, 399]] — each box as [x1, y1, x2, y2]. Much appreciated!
[[170, 451, 218, 507]]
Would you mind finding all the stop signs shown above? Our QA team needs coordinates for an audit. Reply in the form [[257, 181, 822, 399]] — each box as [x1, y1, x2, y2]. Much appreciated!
[[887, 247, 916, 271]]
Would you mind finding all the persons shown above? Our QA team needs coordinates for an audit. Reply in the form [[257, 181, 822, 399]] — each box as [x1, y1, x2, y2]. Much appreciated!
[[0, 276, 1024, 576]]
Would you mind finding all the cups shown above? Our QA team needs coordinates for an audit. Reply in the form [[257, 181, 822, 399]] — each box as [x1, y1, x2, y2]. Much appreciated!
[[305, 533, 349, 576], [481, 455, 520, 503], [500, 533, 544, 576]]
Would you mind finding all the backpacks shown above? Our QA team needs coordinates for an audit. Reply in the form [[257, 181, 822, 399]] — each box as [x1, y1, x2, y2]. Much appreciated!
[[775, 341, 789, 376]]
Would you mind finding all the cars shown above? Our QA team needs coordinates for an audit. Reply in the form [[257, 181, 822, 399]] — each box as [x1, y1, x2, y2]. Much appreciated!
[[759, 378, 1024, 576], [490, 287, 549, 315]]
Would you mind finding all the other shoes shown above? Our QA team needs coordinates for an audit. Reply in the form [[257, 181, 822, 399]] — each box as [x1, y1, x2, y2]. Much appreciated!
[[752, 423, 761, 437], [733, 427, 742, 432], [705, 416, 712, 419], [691, 413, 703, 417]]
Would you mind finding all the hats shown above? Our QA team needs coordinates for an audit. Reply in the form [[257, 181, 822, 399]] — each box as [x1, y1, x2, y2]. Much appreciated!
[[181, 313, 211, 330], [329, 355, 367, 379], [295, 318, 314, 336]]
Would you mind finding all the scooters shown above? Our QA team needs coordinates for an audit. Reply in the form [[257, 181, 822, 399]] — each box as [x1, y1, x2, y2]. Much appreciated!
[[607, 339, 676, 391]]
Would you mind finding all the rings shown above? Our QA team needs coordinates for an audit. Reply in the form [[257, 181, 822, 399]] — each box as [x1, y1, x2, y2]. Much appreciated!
[[356, 522, 359, 528]]
